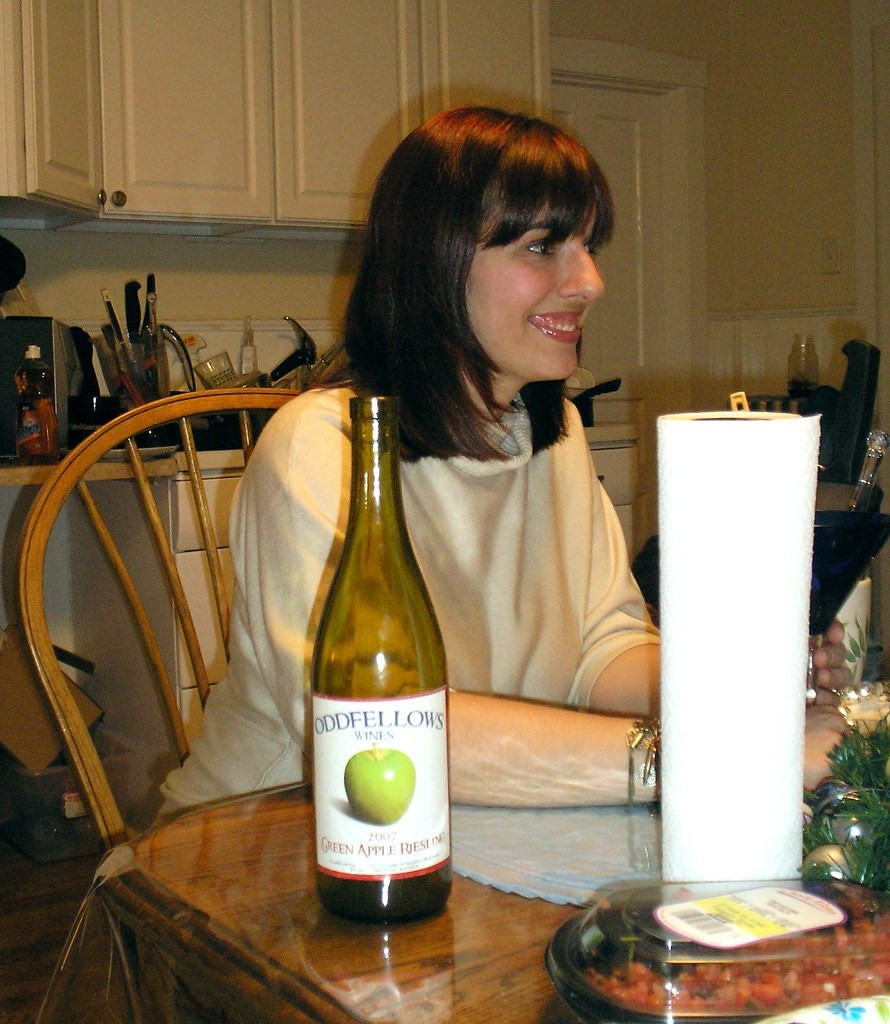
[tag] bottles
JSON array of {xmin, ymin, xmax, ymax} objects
[
  {"xmin": 14, "ymin": 345, "xmax": 59, "ymax": 464},
  {"xmin": 788, "ymin": 333, "xmax": 818, "ymax": 397},
  {"xmin": 312, "ymin": 397, "xmax": 452, "ymax": 926}
]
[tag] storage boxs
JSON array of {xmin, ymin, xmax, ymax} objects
[
  {"xmin": 0, "ymin": 727, "xmax": 136, "ymax": 866},
  {"xmin": 0, "ymin": 625, "xmax": 106, "ymax": 774}
]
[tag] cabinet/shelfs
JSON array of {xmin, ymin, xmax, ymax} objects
[
  {"xmin": 68, "ymin": 441, "xmax": 638, "ymax": 832},
  {"xmin": 1, "ymin": 0, "xmax": 99, "ymax": 231},
  {"xmin": 62, "ymin": 0, "xmax": 553, "ymax": 243}
]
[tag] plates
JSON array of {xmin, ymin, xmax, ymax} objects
[{"xmin": 101, "ymin": 444, "xmax": 180, "ymax": 460}]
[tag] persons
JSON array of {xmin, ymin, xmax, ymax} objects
[{"xmin": 152, "ymin": 105, "xmax": 871, "ymax": 832}]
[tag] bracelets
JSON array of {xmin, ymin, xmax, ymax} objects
[{"xmin": 623, "ymin": 717, "xmax": 664, "ymax": 813}]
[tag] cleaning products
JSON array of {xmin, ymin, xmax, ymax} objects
[{"xmin": 14, "ymin": 345, "xmax": 60, "ymax": 465}]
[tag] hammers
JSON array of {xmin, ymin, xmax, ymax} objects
[{"xmin": 245, "ymin": 316, "xmax": 319, "ymax": 389}]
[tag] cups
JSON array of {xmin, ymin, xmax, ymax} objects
[
  {"xmin": 564, "ymin": 368, "xmax": 595, "ymax": 397},
  {"xmin": 66, "ymin": 395, "xmax": 119, "ymax": 446}
]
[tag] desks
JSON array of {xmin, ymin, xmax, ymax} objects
[{"xmin": 34, "ymin": 782, "xmax": 665, "ymax": 1024}]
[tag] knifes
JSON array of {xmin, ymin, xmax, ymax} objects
[{"xmin": 124, "ymin": 280, "xmax": 141, "ymax": 375}]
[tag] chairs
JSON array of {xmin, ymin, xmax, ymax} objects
[{"xmin": 9, "ymin": 388, "xmax": 316, "ymax": 1024}]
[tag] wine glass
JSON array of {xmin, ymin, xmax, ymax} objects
[{"xmin": 807, "ymin": 510, "xmax": 890, "ymax": 707}]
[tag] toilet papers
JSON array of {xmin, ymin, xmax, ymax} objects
[{"xmin": 655, "ymin": 410, "xmax": 825, "ymax": 884}]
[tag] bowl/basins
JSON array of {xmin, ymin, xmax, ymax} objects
[{"xmin": 546, "ymin": 882, "xmax": 890, "ymax": 1024}]
